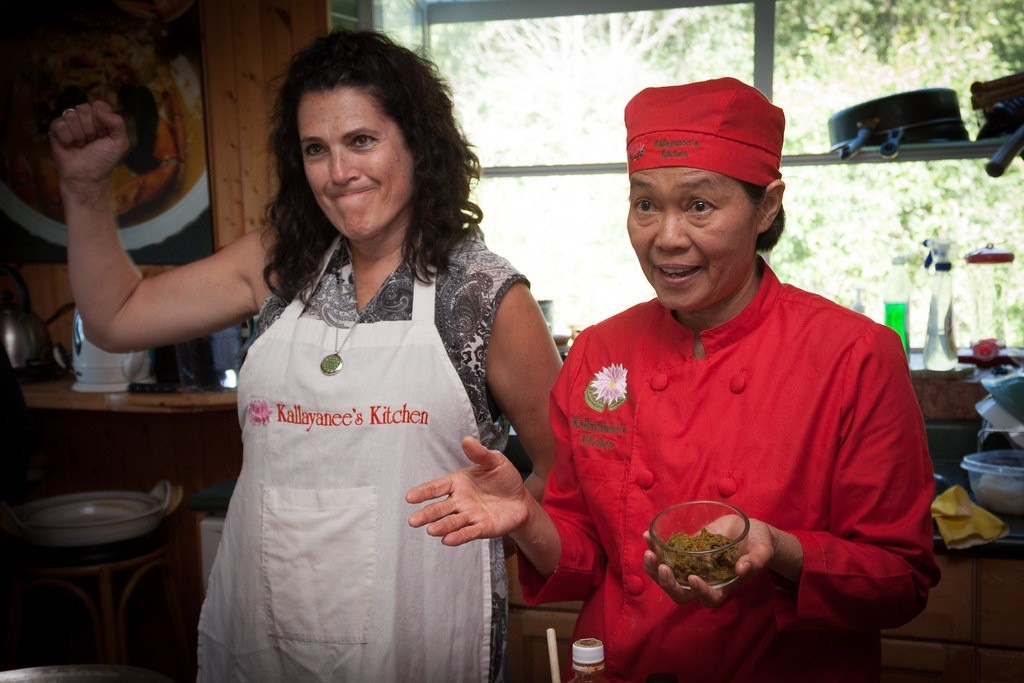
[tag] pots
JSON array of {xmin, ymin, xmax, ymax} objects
[
  {"xmin": 0, "ymin": 480, "xmax": 183, "ymax": 546},
  {"xmin": 830, "ymin": 89, "xmax": 970, "ymax": 163}
]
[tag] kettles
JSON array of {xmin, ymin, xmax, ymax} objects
[{"xmin": 0, "ymin": 263, "xmax": 75, "ymax": 383}]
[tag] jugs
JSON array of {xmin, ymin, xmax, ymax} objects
[{"xmin": 71, "ymin": 273, "xmax": 156, "ymax": 393}]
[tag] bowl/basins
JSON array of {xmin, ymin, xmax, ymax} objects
[
  {"xmin": 650, "ymin": 501, "xmax": 750, "ymax": 590},
  {"xmin": 959, "ymin": 447, "xmax": 1024, "ymax": 516}
]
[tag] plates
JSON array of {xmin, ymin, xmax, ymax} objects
[{"xmin": 2, "ymin": 21, "xmax": 210, "ymax": 250}]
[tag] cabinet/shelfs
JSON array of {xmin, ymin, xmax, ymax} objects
[{"xmin": 192, "ymin": 511, "xmax": 1024, "ymax": 683}]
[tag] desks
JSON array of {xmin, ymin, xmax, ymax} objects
[{"xmin": 20, "ymin": 383, "xmax": 238, "ymax": 415}]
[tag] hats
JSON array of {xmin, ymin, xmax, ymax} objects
[{"xmin": 624, "ymin": 77, "xmax": 785, "ymax": 188}]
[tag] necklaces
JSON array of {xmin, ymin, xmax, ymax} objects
[{"xmin": 319, "ymin": 238, "xmax": 404, "ymax": 377}]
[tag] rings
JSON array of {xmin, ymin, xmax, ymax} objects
[{"xmin": 62, "ymin": 109, "xmax": 75, "ymax": 120}]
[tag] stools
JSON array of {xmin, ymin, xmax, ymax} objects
[{"xmin": 8, "ymin": 530, "xmax": 187, "ymax": 666}]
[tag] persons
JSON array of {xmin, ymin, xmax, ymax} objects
[
  {"xmin": 404, "ymin": 76, "xmax": 942, "ymax": 683},
  {"xmin": 46, "ymin": 25, "xmax": 563, "ymax": 683}
]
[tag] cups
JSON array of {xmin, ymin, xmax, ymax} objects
[{"xmin": 176, "ymin": 316, "xmax": 259, "ymax": 392}]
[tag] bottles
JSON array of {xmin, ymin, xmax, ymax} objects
[
  {"xmin": 884, "ymin": 235, "xmax": 1022, "ymax": 372},
  {"xmin": 566, "ymin": 637, "xmax": 612, "ymax": 683}
]
